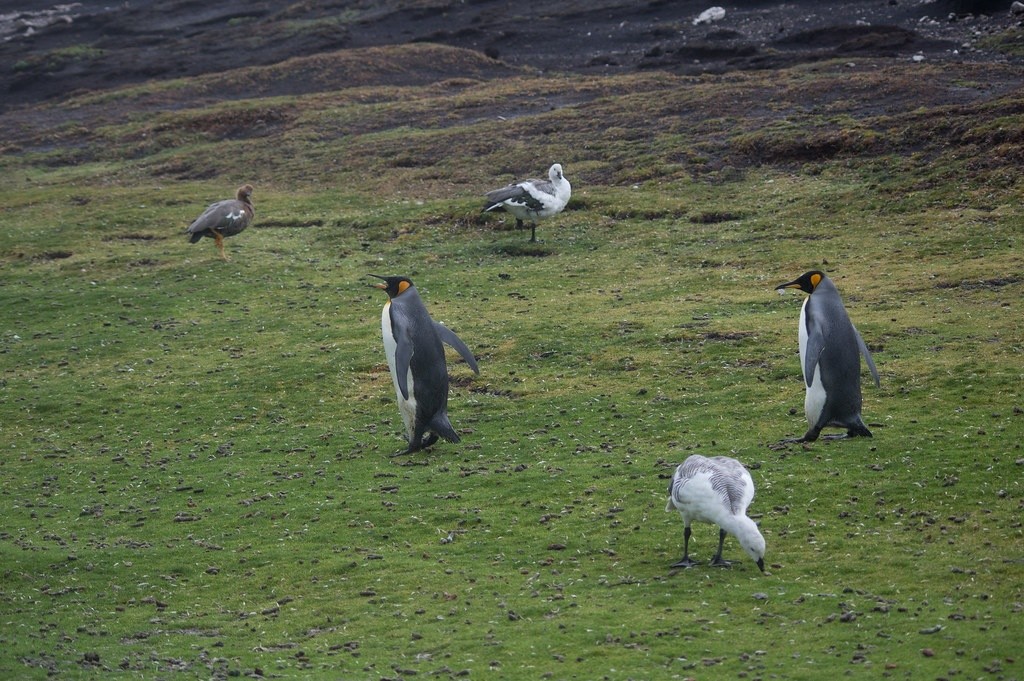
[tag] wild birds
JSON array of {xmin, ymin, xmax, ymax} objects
[
  {"xmin": 664, "ymin": 453, "xmax": 767, "ymax": 574},
  {"xmin": 186, "ymin": 184, "xmax": 255, "ymax": 263},
  {"xmin": 480, "ymin": 164, "xmax": 571, "ymax": 243}
]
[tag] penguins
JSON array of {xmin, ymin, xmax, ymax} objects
[
  {"xmin": 774, "ymin": 270, "xmax": 881, "ymax": 444},
  {"xmin": 361, "ymin": 270, "xmax": 482, "ymax": 456}
]
